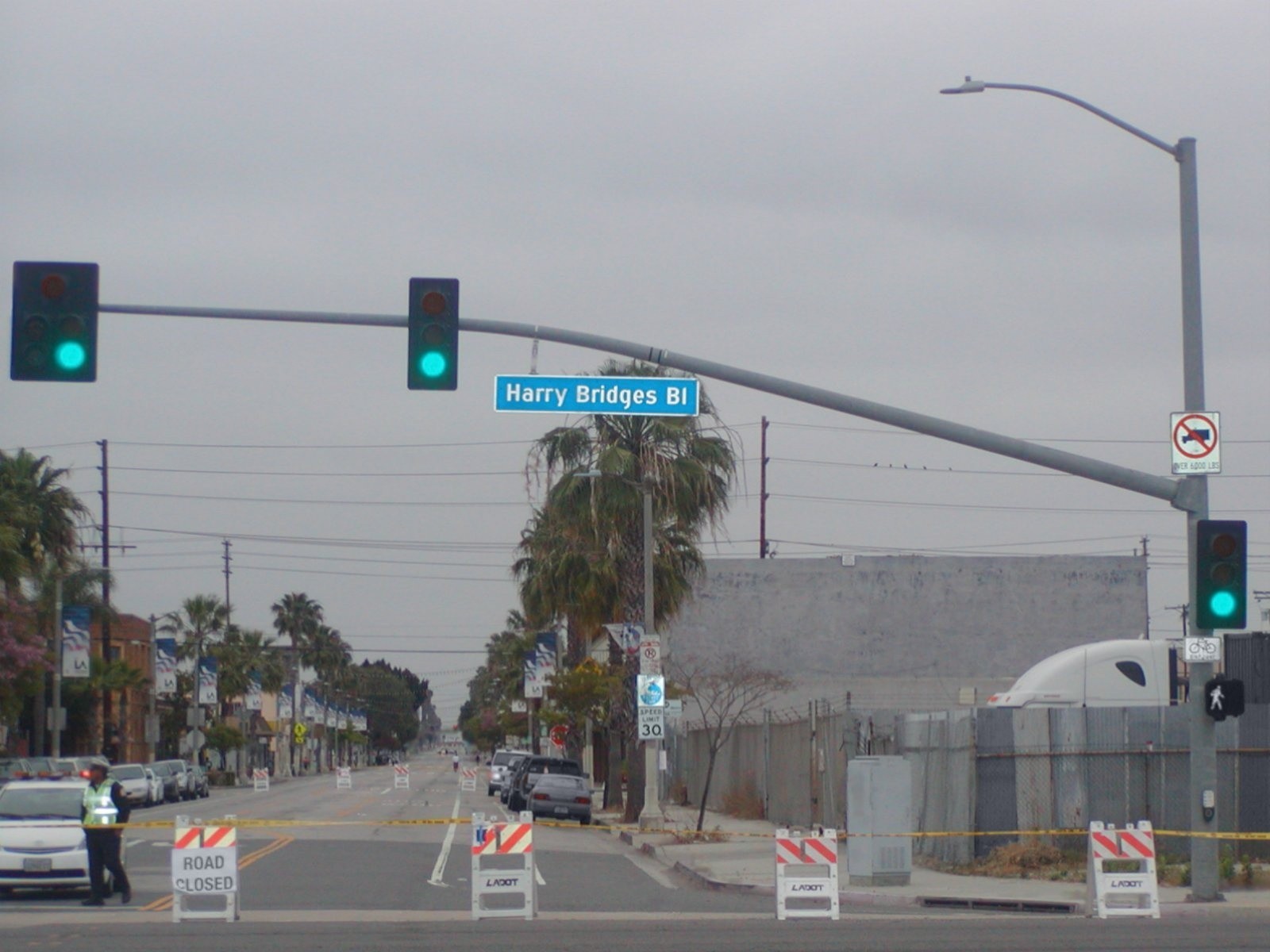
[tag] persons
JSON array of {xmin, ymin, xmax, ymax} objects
[
  {"xmin": 453, "ymin": 753, "xmax": 458, "ymax": 772},
  {"xmin": 303, "ymin": 756, "xmax": 308, "ymax": 769},
  {"xmin": 476, "ymin": 755, "xmax": 479, "ymax": 766},
  {"xmin": 81, "ymin": 759, "xmax": 130, "ymax": 905},
  {"xmin": 204, "ymin": 758, "xmax": 212, "ymax": 769}
]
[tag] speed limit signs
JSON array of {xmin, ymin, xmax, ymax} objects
[{"xmin": 638, "ymin": 707, "xmax": 664, "ymax": 741}]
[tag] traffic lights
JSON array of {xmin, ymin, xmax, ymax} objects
[
  {"xmin": 10, "ymin": 261, "xmax": 99, "ymax": 382},
  {"xmin": 1206, "ymin": 673, "xmax": 1245, "ymax": 721},
  {"xmin": 1196, "ymin": 518, "xmax": 1249, "ymax": 629},
  {"xmin": 409, "ymin": 278, "xmax": 457, "ymax": 390}
]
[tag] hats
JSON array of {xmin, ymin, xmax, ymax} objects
[{"xmin": 84, "ymin": 755, "xmax": 111, "ymax": 770}]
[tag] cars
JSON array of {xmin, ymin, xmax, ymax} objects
[
  {"xmin": 0, "ymin": 770, "xmax": 128, "ymax": 898},
  {"xmin": 485, "ymin": 746, "xmax": 595, "ymax": 826},
  {"xmin": 0, "ymin": 754, "xmax": 211, "ymax": 809}
]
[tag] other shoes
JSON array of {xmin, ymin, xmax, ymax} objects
[
  {"xmin": 122, "ymin": 886, "xmax": 131, "ymax": 904},
  {"xmin": 81, "ymin": 895, "xmax": 105, "ymax": 906}
]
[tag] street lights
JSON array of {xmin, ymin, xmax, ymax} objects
[{"xmin": 944, "ymin": 73, "xmax": 1224, "ymax": 903}]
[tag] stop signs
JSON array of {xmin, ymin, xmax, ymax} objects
[{"xmin": 550, "ymin": 724, "xmax": 569, "ymax": 746}]
